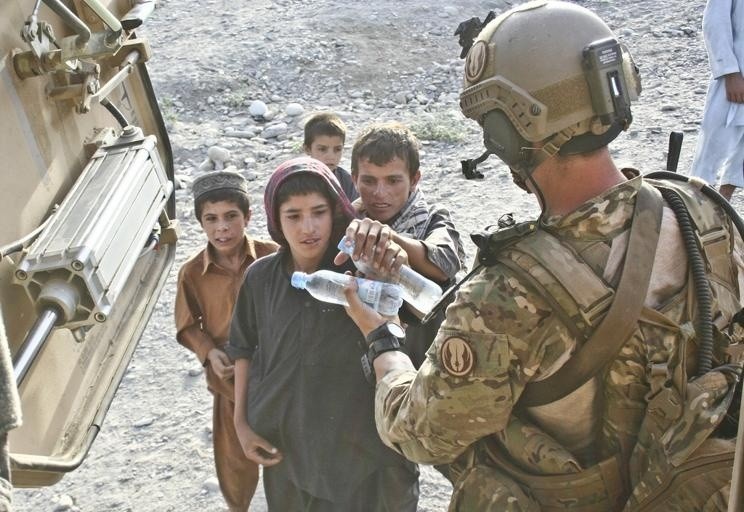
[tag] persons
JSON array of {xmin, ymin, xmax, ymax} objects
[
  {"xmin": 224, "ymin": 155, "xmax": 419, "ymax": 511},
  {"xmin": 173, "ymin": 169, "xmax": 278, "ymax": 512},
  {"xmin": 686, "ymin": 0, "xmax": 744, "ymax": 202},
  {"xmin": 339, "ymin": 0, "xmax": 743, "ymax": 512},
  {"xmin": 334, "ymin": 121, "xmax": 467, "ymax": 371},
  {"xmin": 301, "ymin": 109, "xmax": 361, "ymax": 203}
]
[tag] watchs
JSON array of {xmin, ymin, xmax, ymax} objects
[
  {"xmin": 366, "ymin": 321, "xmax": 407, "ymax": 345},
  {"xmin": 360, "ymin": 335, "xmax": 401, "ymax": 387}
]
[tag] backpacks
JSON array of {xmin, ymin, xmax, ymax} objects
[{"xmin": 621, "ymin": 363, "xmax": 744, "ymax": 512}]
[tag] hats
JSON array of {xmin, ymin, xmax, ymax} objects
[{"xmin": 192, "ymin": 171, "xmax": 248, "ymax": 199}]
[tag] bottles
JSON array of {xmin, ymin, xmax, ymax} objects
[
  {"xmin": 290, "ymin": 270, "xmax": 404, "ymax": 317},
  {"xmin": 336, "ymin": 236, "xmax": 442, "ymax": 314}
]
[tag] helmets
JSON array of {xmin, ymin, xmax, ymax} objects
[{"xmin": 455, "ymin": 0, "xmax": 641, "ymax": 143}]
